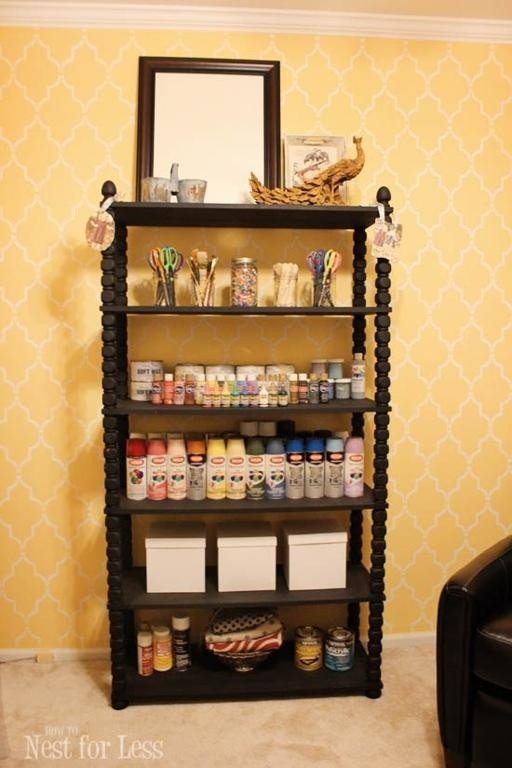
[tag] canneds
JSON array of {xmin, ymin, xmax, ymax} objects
[
  {"xmin": 130, "ymin": 381, "xmax": 153, "ymax": 402},
  {"xmin": 324, "ymin": 626, "xmax": 354, "ymax": 672},
  {"xmin": 130, "ymin": 359, "xmax": 163, "ymax": 381},
  {"xmin": 294, "ymin": 627, "xmax": 324, "ymax": 672}
]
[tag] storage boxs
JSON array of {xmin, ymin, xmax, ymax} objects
[
  {"xmin": 216, "ymin": 520, "xmax": 278, "ymax": 593},
  {"xmin": 279, "ymin": 519, "xmax": 349, "ymax": 591},
  {"xmin": 143, "ymin": 520, "xmax": 207, "ymax": 594}
]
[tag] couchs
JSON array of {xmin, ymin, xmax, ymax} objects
[
  {"xmin": 436, "ymin": 534, "xmax": 512, "ymax": 768},
  {"xmin": 134, "ymin": 56, "xmax": 281, "ymax": 204}
]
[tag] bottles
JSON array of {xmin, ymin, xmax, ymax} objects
[
  {"xmin": 206, "ymin": 373, "xmax": 217, "ymax": 403},
  {"xmin": 327, "ymin": 358, "xmax": 345, "ymax": 378},
  {"xmin": 174, "ymin": 361, "xmax": 204, "ymax": 381},
  {"xmin": 205, "ymin": 363, "xmax": 235, "ymax": 373},
  {"xmin": 288, "ymin": 373, "xmax": 298, "ymax": 404},
  {"xmin": 257, "ymin": 373, "xmax": 267, "ymax": 404},
  {"xmin": 152, "ymin": 626, "xmax": 172, "ymax": 673},
  {"xmin": 309, "ymin": 373, "xmax": 319, "ymax": 403},
  {"xmin": 236, "ymin": 373, "xmax": 247, "ymax": 404},
  {"xmin": 278, "ymin": 384, "xmax": 289, "ymax": 406},
  {"xmin": 222, "ymin": 381, "xmax": 230, "ymax": 407},
  {"xmin": 351, "ymin": 352, "xmax": 366, "ymax": 398},
  {"xmin": 226, "ymin": 373, "xmax": 236, "ymax": 404},
  {"xmin": 195, "ymin": 373, "xmax": 205, "ymax": 404},
  {"xmin": 185, "ymin": 373, "xmax": 195, "ymax": 404},
  {"xmin": 311, "ymin": 358, "xmax": 328, "ymax": 377},
  {"xmin": 217, "ymin": 373, "xmax": 226, "ymax": 404},
  {"xmin": 298, "ymin": 373, "xmax": 309, "ymax": 404},
  {"xmin": 259, "ymin": 386, "xmax": 269, "ymax": 407},
  {"xmin": 266, "ymin": 362, "xmax": 296, "ymax": 373},
  {"xmin": 279, "ymin": 372, "xmax": 290, "ymax": 402},
  {"xmin": 235, "ymin": 363, "xmax": 266, "ymax": 374},
  {"xmin": 231, "ymin": 386, "xmax": 240, "ymax": 406},
  {"xmin": 241, "ymin": 385, "xmax": 249, "ymax": 406},
  {"xmin": 267, "ymin": 373, "xmax": 279, "ymax": 404},
  {"xmin": 335, "ymin": 378, "xmax": 351, "ymax": 398},
  {"xmin": 318, "ymin": 373, "xmax": 330, "ymax": 403},
  {"xmin": 203, "ymin": 382, "xmax": 211, "ymax": 407},
  {"xmin": 230, "ymin": 257, "xmax": 258, "ymax": 305},
  {"xmin": 247, "ymin": 373, "xmax": 257, "ymax": 404},
  {"xmin": 250, "ymin": 386, "xmax": 259, "ymax": 407},
  {"xmin": 327, "ymin": 378, "xmax": 335, "ymax": 398},
  {"xmin": 212, "ymin": 381, "xmax": 221, "ymax": 407},
  {"xmin": 171, "ymin": 613, "xmax": 191, "ymax": 672},
  {"xmin": 174, "ymin": 373, "xmax": 184, "ymax": 404},
  {"xmin": 152, "ymin": 373, "xmax": 163, "ymax": 403},
  {"xmin": 269, "ymin": 385, "xmax": 278, "ymax": 406},
  {"xmin": 164, "ymin": 373, "xmax": 173, "ymax": 404}
]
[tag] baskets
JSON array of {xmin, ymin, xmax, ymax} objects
[{"xmin": 202, "ymin": 608, "xmax": 275, "ymax": 672}]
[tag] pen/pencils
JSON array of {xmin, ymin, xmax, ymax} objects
[{"xmin": 186, "ymin": 249, "xmax": 221, "ymax": 307}]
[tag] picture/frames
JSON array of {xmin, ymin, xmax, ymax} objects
[{"xmin": 281, "ymin": 133, "xmax": 348, "ymax": 206}]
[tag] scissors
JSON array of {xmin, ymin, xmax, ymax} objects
[
  {"xmin": 147, "ymin": 246, "xmax": 184, "ymax": 306},
  {"xmin": 305, "ymin": 248, "xmax": 343, "ymax": 307}
]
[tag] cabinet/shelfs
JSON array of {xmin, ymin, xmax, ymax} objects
[{"xmin": 99, "ymin": 180, "xmax": 393, "ymax": 710}]
